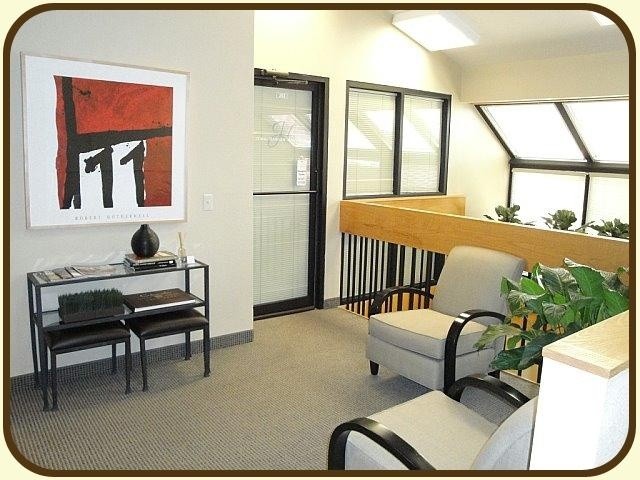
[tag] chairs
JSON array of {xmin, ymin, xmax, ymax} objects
[
  {"xmin": 328, "ymin": 372, "xmax": 539, "ymax": 471},
  {"xmin": 367, "ymin": 242, "xmax": 524, "ymax": 391}
]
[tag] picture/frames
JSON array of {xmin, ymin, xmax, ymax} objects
[{"xmin": 20, "ymin": 51, "xmax": 190, "ymax": 231}]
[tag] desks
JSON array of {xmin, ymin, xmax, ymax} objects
[{"xmin": 27, "ymin": 258, "xmax": 218, "ymax": 411}]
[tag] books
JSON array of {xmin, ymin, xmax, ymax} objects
[
  {"xmin": 121, "ymin": 288, "xmax": 197, "ymax": 313},
  {"xmin": 33, "ymin": 249, "xmax": 179, "ymax": 284}
]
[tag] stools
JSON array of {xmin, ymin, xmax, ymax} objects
[{"xmin": 43, "ymin": 309, "xmax": 209, "ymax": 411}]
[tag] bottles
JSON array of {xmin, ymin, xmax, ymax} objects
[{"xmin": 176, "ymin": 232, "xmax": 188, "ymax": 267}]
